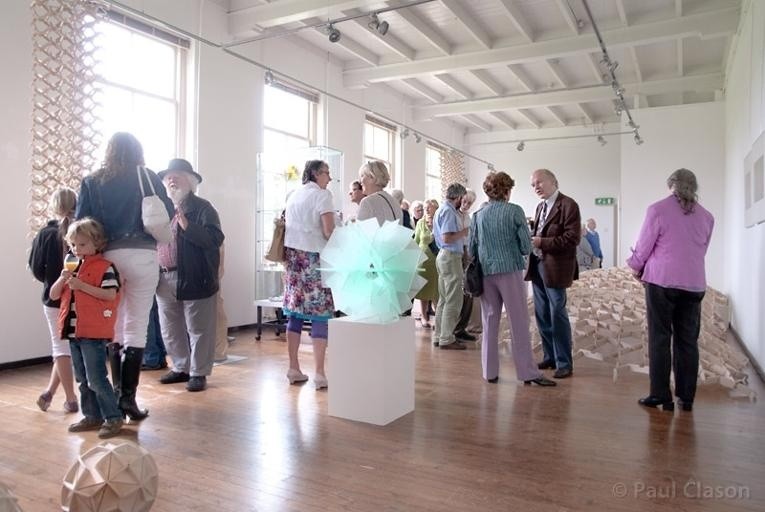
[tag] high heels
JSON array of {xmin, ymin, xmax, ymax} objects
[
  {"xmin": 314, "ymin": 373, "xmax": 328, "ymax": 390},
  {"xmin": 287, "ymin": 369, "xmax": 308, "ymax": 384},
  {"xmin": 677, "ymin": 398, "xmax": 693, "ymax": 411},
  {"xmin": 639, "ymin": 396, "xmax": 674, "ymax": 411}
]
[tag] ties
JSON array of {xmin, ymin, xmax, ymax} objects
[{"xmin": 533, "ymin": 202, "xmax": 547, "ymax": 260}]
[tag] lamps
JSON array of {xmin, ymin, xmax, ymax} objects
[
  {"xmin": 328, "ymin": 13, "xmax": 389, "ymax": 42},
  {"xmin": 605, "ymin": 60, "xmax": 644, "ymax": 145},
  {"xmin": 517, "ymin": 142, "xmax": 525, "ymax": 151},
  {"xmin": 400, "ymin": 129, "xmax": 496, "ymax": 174},
  {"xmin": 598, "ymin": 136, "xmax": 605, "ymax": 144}
]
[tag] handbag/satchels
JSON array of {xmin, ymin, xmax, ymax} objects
[
  {"xmin": 266, "ymin": 218, "xmax": 285, "ymax": 261},
  {"xmin": 141, "ymin": 196, "xmax": 172, "ymax": 242},
  {"xmin": 464, "ymin": 262, "xmax": 484, "ymax": 297}
]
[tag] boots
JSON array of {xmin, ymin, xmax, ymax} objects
[
  {"xmin": 108, "ymin": 342, "xmax": 124, "ymax": 400},
  {"xmin": 117, "ymin": 346, "xmax": 148, "ymax": 420}
]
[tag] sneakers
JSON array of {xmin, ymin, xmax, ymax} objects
[{"xmin": 38, "ymin": 362, "xmax": 206, "ymax": 438}]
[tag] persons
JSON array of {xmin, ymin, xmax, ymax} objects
[
  {"xmin": 47, "ymin": 215, "xmax": 125, "ymax": 438},
  {"xmin": 575, "ymin": 224, "xmax": 601, "ymax": 274},
  {"xmin": 138, "ymin": 158, "xmax": 229, "ymax": 393},
  {"xmin": 584, "ymin": 218, "xmax": 603, "ymax": 268},
  {"xmin": 523, "ymin": 167, "xmax": 582, "ymax": 380},
  {"xmin": 281, "ymin": 157, "xmax": 337, "ymax": 391},
  {"xmin": 26, "ymin": 188, "xmax": 79, "ymax": 413},
  {"xmin": 75, "ymin": 130, "xmax": 176, "ymax": 421},
  {"xmin": 466, "ymin": 170, "xmax": 557, "ymax": 388},
  {"xmin": 347, "ymin": 159, "xmax": 483, "ymax": 351},
  {"xmin": 625, "ymin": 165, "xmax": 714, "ymax": 413}
]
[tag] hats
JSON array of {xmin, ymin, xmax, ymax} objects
[{"xmin": 157, "ymin": 159, "xmax": 202, "ymax": 183}]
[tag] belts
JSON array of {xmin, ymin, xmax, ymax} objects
[{"xmin": 160, "ymin": 265, "xmax": 177, "ymax": 273}]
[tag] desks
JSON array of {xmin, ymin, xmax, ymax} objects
[{"xmin": 253, "ymin": 296, "xmax": 340, "ymax": 340}]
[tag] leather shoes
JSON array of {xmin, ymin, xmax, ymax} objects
[{"xmin": 402, "ymin": 311, "xmax": 571, "ymax": 386}]
